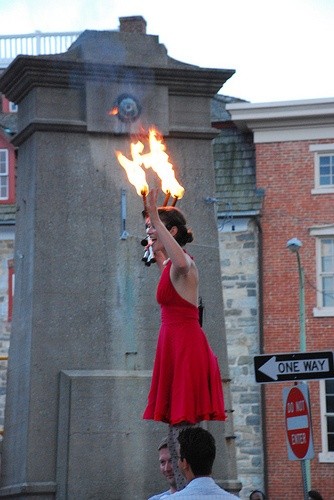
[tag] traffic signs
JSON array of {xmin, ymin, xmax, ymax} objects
[{"xmin": 253, "ymin": 350, "xmax": 334, "ymax": 383}]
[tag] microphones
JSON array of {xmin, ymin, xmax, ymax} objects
[{"xmin": 141, "ymin": 236, "xmax": 149, "ymax": 246}]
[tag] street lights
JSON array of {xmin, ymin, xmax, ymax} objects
[{"xmin": 283, "ymin": 236, "xmax": 314, "ymax": 500}]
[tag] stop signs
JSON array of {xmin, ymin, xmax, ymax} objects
[{"xmin": 281, "ymin": 383, "xmax": 315, "ymax": 460}]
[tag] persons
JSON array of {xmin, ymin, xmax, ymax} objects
[
  {"xmin": 147, "ymin": 427, "xmax": 324, "ymax": 500},
  {"xmin": 141, "ymin": 189, "xmax": 225, "ymax": 491}
]
